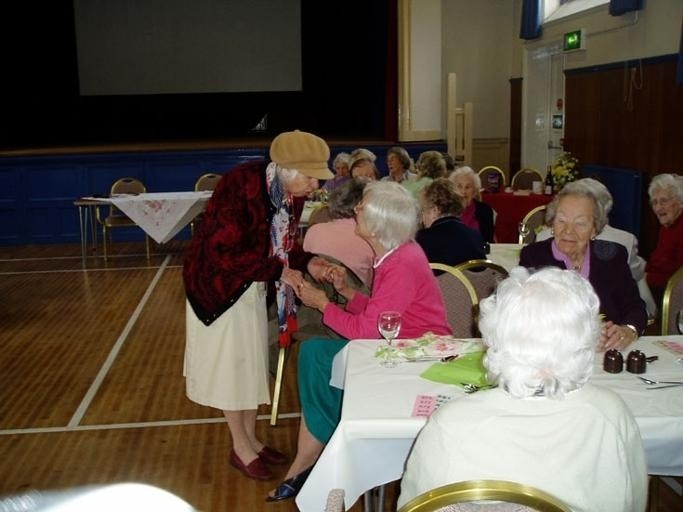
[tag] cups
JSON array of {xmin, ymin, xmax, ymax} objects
[
  {"xmin": 604, "ymin": 349, "xmax": 622, "ymax": 374},
  {"xmin": 627, "ymin": 350, "xmax": 646, "ymax": 374},
  {"xmin": 533, "ymin": 181, "xmax": 544, "ymax": 195}
]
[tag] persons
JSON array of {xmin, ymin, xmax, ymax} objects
[
  {"xmin": 265, "ymin": 147, "xmax": 682, "ymax": 512},
  {"xmin": 183, "ymin": 129, "xmax": 336, "ymax": 481}
]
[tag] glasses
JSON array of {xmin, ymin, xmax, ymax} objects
[
  {"xmin": 353, "ymin": 200, "xmax": 363, "ymax": 214},
  {"xmin": 650, "ymin": 195, "xmax": 677, "ymax": 205}
]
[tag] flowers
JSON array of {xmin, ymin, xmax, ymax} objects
[{"xmin": 550, "ymin": 151, "xmax": 579, "ymax": 191}]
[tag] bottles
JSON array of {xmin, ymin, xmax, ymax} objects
[{"xmin": 544, "ymin": 165, "xmax": 554, "ymax": 195}]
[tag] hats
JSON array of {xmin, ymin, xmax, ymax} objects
[
  {"xmin": 269, "ymin": 130, "xmax": 335, "ymax": 180},
  {"xmin": 349, "ymin": 148, "xmax": 376, "ymax": 170}
]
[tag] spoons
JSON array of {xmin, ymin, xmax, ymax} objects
[
  {"xmin": 636, "ymin": 376, "xmax": 683, "ymax": 387},
  {"xmin": 458, "ymin": 383, "xmax": 498, "ymax": 393},
  {"xmin": 405, "ymin": 353, "xmax": 458, "ymax": 362}
]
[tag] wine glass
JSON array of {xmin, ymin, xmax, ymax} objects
[{"xmin": 376, "ymin": 312, "xmax": 402, "ymax": 368}]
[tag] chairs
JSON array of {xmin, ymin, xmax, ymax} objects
[
  {"xmin": 191, "ymin": 173, "xmax": 224, "ymax": 237},
  {"xmin": 96, "ymin": 177, "xmax": 150, "ymax": 261}
]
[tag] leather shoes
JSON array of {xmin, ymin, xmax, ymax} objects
[
  {"xmin": 265, "ymin": 463, "xmax": 315, "ymax": 502},
  {"xmin": 257, "ymin": 444, "xmax": 289, "ymax": 465},
  {"xmin": 228, "ymin": 447, "xmax": 277, "ymax": 482}
]
